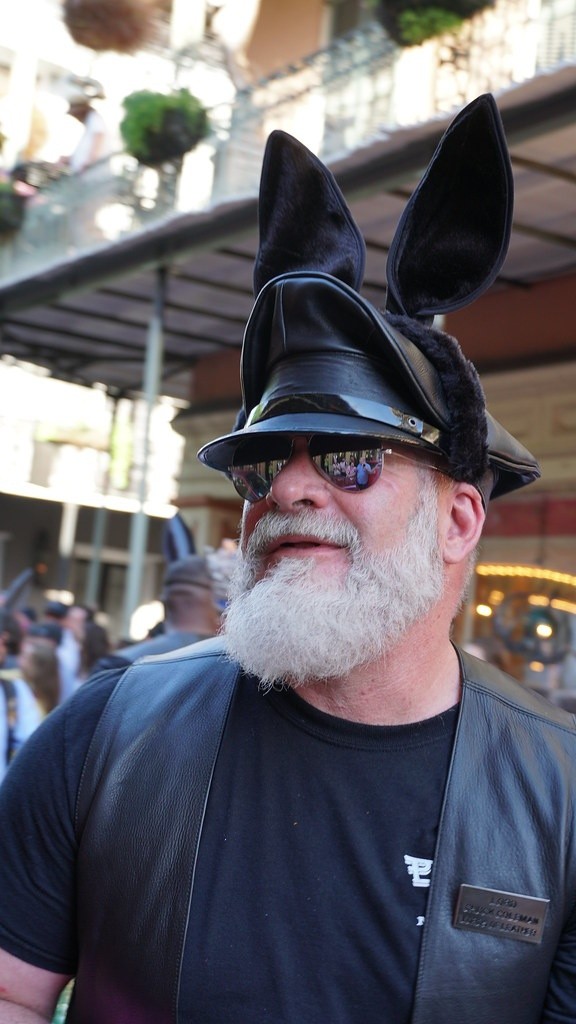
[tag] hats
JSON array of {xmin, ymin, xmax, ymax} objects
[{"xmin": 196, "ymin": 270, "xmax": 542, "ymax": 500}]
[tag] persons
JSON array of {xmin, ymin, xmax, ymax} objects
[
  {"xmin": 89, "ymin": 553, "xmax": 231, "ymax": 676},
  {"xmin": 0, "ymin": 92, "xmax": 576, "ymax": 1024},
  {"xmin": 0, "ymin": 600, "xmax": 108, "ymax": 773},
  {"xmin": 333, "ymin": 455, "xmax": 356, "ymax": 479},
  {"xmin": 357, "ymin": 456, "xmax": 371, "ymax": 490}
]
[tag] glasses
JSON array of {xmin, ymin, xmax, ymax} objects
[{"xmin": 226, "ymin": 436, "xmax": 447, "ymax": 504}]
